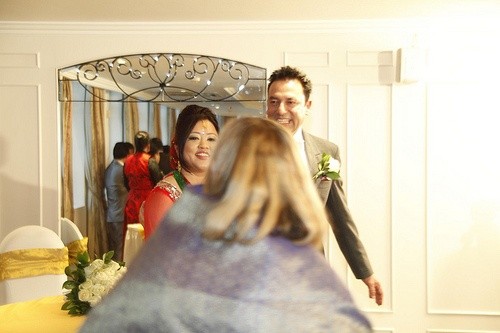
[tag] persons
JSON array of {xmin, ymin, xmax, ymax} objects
[
  {"xmin": 103, "ymin": 131, "xmax": 176, "ymax": 263},
  {"xmin": 143, "ymin": 104, "xmax": 221, "ymax": 241},
  {"xmin": 265, "ymin": 66, "xmax": 384, "ymax": 306},
  {"xmin": 75, "ymin": 116, "xmax": 375, "ymax": 333}
]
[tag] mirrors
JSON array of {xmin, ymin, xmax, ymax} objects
[{"xmin": 58, "ymin": 53, "xmax": 267, "ymax": 262}]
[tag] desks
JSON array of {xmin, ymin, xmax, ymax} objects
[{"xmin": 123, "ymin": 223, "xmax": 145, "ymax": 267}]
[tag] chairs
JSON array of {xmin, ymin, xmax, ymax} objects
[
  {"xmin": 61, "ymin": 218, "xmax": 90, "ymax": 264},
  {"xmin": 0, "ymin": 225, "xmax": 72, "ymax": 306}
]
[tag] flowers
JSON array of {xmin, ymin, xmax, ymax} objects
[
  {"xmin": 311, "ymin": 152, "xmax": 341, "ymax": 183},
  {"xmin": 61, "ymin": 248, "xmax": 128, "ymax": 314}
]
